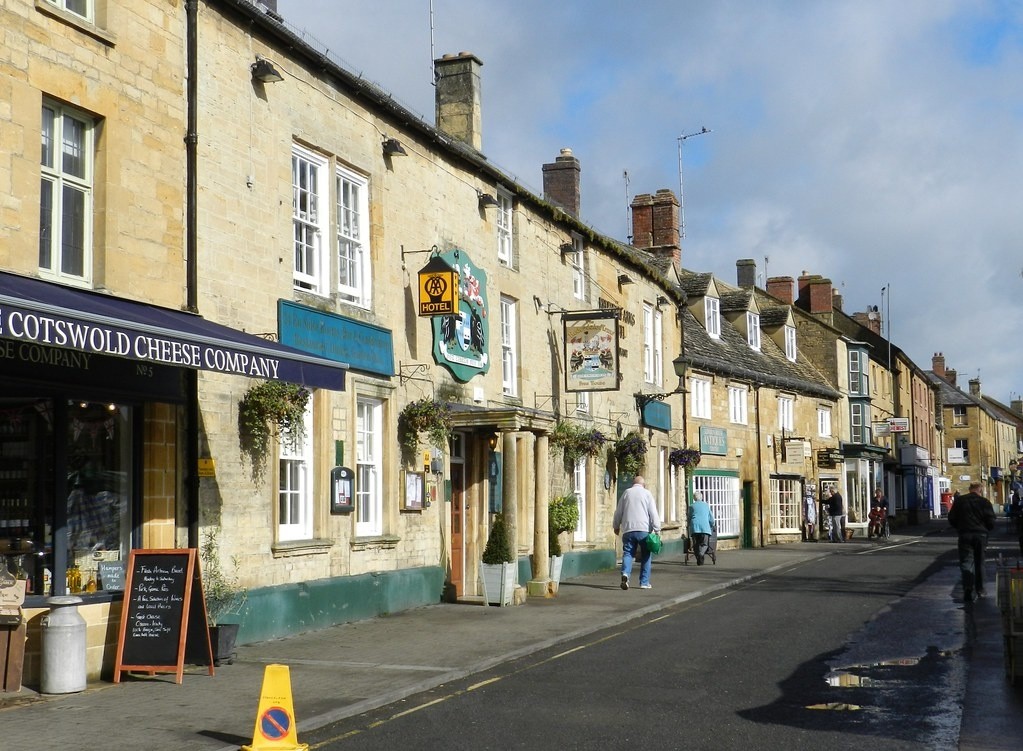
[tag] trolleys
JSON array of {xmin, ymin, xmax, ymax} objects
[{"xmin": 685, "ymin": 525, "xmax": 719, "ymax": 567}]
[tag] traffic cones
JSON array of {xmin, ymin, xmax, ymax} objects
[{"xmin": 238, "ymin": 662, "xmax": 308, "ymax": 751}]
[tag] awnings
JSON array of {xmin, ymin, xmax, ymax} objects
[{"xmin": 0, "ymin": 268, "xmax": 350, "ymax": 394}]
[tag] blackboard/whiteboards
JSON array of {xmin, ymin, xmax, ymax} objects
[
  {"xmin": 115, "ymin": 548, "xmax": 213, "ymax": 671},
  {"xmin": 98, "ymin": 562, "xmax": 126, "ymax": 590}
]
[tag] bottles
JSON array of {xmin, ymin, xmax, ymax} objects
[
  {"xmin": 1, "ymin": 408, "xmax": 32, "ymax": 552},
  {"xmin": 1, "ymin": 557, "xmax": 26, "ymax": 580},
  {"xmin": 66, "ymin": 565, "xmax": 96, "ymax": 594}
]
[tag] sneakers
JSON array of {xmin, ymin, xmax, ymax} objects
[
  {"xmin": 620, "ymin": 574, "xmax": 629, "ymax": 590},
  {"xmin": 640, "ymin": 584, "xmax": 652, "ymax": 588}
]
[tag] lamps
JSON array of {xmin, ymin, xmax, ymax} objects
[
  {"xmin": 252, "ymin": 60, "xmax": 283, "ymax": 82},
  {"xmin": 561, "ymin": 243, "xmax": 576, "ymax": 254},
  {"xmin": 619, "ymin": 275, "xmax": 635, "ymax": 285},
  {"xmin": 634, "ymin": 353, "xmax": 691, "ymax": 408},
  {"xmin": 382, "ymin": 139, "xmax": 407, "ymax": 156},
  {"xmin": 478, "ymin": 194, "xmax": 500, "ymax": 208},
  {"xmin": 657, "ymin": 296, "xmax": 669, "ymax": 306}
]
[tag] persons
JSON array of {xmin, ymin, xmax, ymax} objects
[
  {"xmin": 686, "ymin": 490, "xmax": 714, "ymax": 566},
  {"xmin": 813, "ymin": 484, "xmax": 846, "ymax": 545},
  {"xmin": 952, "ymin": 489, "xmax": 961, "ymax": 500},
  {"xmin": 867, "ymin": 487, "xmax": 890, "ymax": 538},
  {"xmin": 948, "ymin": 483, "xmax": 996, "ymax": 609},
  {"xmin": 612, "ymin": 476, "xmax": 660, "ymax": 590},
  {"xmin": 1008, "ymin": 490, "xmax": 1014, "ymax": 505}
]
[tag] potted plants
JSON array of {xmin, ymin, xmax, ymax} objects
[
  {"xmin": 670, "ymin": 447, "xmax": 701, "ymax": 476},
  {"xmin": 614, "ymin": 430, "xmax": 650, "ymax": 474},
  {"xmin": 550, "ymin": 422, "xmax": 604, "ymax": 464},
  {"xmin": 398, "ymin": 398, "xmax": 457, "ymax": 454},
  {"xmin": 480, "ymin": 514, "xmax": 516, "ymax": 608},
  {"xmin": 530, "ymin": 493, "xmax": 580, "ymax": 591},
  {"xmin": 198, "ymin": 500, "xmax": 248, "ymax": 659},
  {"xmin": 241, "ymin": 379, "xmax": 311, "ymax": 455}
]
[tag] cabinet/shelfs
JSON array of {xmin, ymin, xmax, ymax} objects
[{"xmin": 0, "ymin": 395, "xmax": 54, "ymax": 554}]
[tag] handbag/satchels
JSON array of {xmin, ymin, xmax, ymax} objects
[{"xmin": 646, "ymin": 533, "xmax": 662, "ymax": 554}]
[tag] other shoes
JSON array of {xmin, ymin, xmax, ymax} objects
[
  {"xmin": 978, "ymin": 590, "xmax": 988, "ymax": 597},
  {"xmin": 964, "ymin": 586, "xmax": 973, "ymax": 601},
  {"xmin": 697, "ymin": 554, "xmax": 704, "ymax": 565}
]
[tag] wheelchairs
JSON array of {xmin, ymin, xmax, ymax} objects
[{"xmin": 868, "ymin": 507, "xmax": 890, "ymax": 540}]
